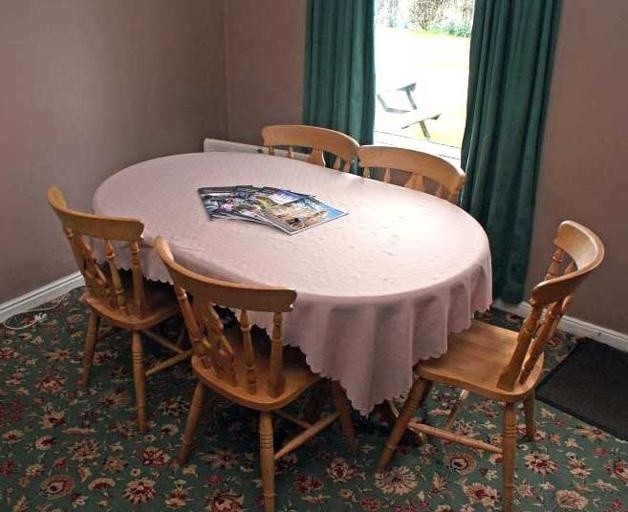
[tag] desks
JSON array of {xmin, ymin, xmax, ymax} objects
[{"xmin": 92, "ymin": 151, "xmax": 493, "ymax": 447}]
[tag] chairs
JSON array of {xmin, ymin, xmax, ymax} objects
[
  {"xmin": 358, "ymin": 144, "xmax": 466, "ymax": 202},
  {"xmin": 154, "ymin": 236, "xmax": 356, "ymax": 512},
  {"xmin": 46, "ymin": 185, "xmax": 193, "ymax": 435},
  {"xmin": 261, "ymin": 124, "xmax": 360, "ymax": 173},
  {"xmin": 377, "ymin": 220, "xmax": 606, "ymax": 512}
]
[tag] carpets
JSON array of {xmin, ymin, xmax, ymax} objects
[{"xmin": 534, "ymin": 336, "xmax": 628, "ymax": 442}]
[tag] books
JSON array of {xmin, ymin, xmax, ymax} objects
[{"xmin": 198, "ymin": 184, "xmax": 348, "ymax": 235}]
